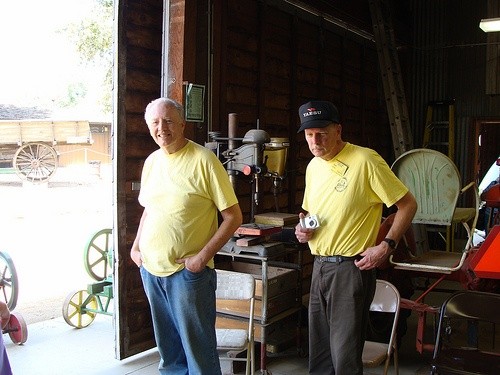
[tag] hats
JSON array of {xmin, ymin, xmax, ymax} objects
[{"xmin": 297, "ymin": 101, "xmax": 339, "ymax": 133}]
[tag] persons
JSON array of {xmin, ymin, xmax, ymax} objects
[
  {"xmin": 128, "ymin": 98, "xmax": 243, "ymax": 375},
  {"xmin": 295, "ymin": 100, "xmax": 418, "ymax": 375}
]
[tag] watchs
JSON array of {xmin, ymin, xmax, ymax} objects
[{"xmin": 384, "ymin": 238, "xmax": 396, "ymax": 249}]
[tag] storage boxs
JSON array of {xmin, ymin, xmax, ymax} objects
[{"xmin": 215, "ymin": 260, "xmax": 298, "ymax": 343}]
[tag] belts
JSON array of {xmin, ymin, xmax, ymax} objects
[{"xmin": 315, "ymin": 256, "xmax": 353, "ymax": 263}]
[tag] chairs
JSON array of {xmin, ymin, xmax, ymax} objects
[
  {"xmin": 430, "ymin": 290, "xmax": 500, "ymax": 375},
  {"xmin": 389, "ymin": 148, "xmax": 481, "ymax": 271},
  {"xmin": 212, "ymin": 269, "xmax": 256, "ymax": 375},
  {"xmin": 362, "ymin": 279, "xmax": 401, "ymax": 375}
]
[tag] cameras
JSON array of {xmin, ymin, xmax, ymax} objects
[{"xmin": 299, "ymin": 214, "xmax": 320, "ymax": 230}]
